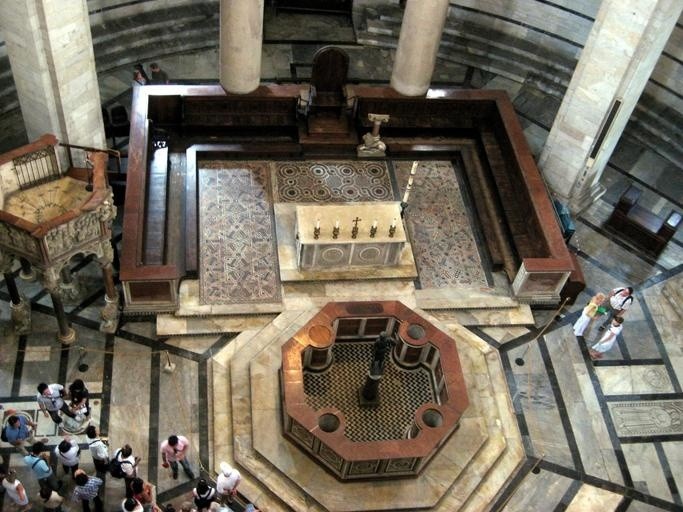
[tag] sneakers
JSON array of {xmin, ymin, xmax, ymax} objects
[
  {"xmin": 75, "ymin": 416, "xmax": 81, "ymax": 421},
  {"xmin": 59, "ymin": 422, "xmax": 64, "ymax": 428}
]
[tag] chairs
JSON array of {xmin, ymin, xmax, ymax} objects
[{"xmin": 297, "ymin": 46, "xmax": 358, "ymax": 137}]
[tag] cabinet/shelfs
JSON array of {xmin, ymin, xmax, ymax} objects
[
  {"xmin": 560, "ymin": 248, "xmax": 585, "ymax": 305},
  {"xmin": 601, "ymin": 184, "xmax": 683, "ymax": 260}
]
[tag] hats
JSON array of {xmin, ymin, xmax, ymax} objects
[
  {"xmin": 220, "ymin": 462, "xmax": 232, "ymax": 477},
  {"xmin": 182, "ymin": 502, "xmax": 192, "ymax": 510}
]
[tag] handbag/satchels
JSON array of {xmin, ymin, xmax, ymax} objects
[{"xmin": 76, "ymin": 404, "xmax": 87, "ymax": 414}]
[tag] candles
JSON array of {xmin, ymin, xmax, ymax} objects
[
  {"xmin": 392, "ymin": 217, "xmax": 398, "ymax": 228},
  {"xmin": 316, "ymin": 219, "xmax": 320, "ymax": 230},
  {"xmin": 373, "ymin": 219, "xmax": 378, "ymax": 228},
  {"xmin": 335, "ymin": 220, "xmax": 339, "ymax": 228}
]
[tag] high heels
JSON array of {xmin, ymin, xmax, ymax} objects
[{"xmin": 23, "ymin": 503, "xmax": 32, "ymax": 512}]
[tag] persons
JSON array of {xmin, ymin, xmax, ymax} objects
[
  {"xmin": 0, "ymin": 377, "xmax": 261, "ymax": 512},
  {"xmin": 131, "ymin": 62, "xmax": 151, "ymax": 88},
  {"xmin": 146, "ymin": 62, "xmax": 169, "ymax": 85},
  {"xmin": 594, "ymin": 287, "xmax": 634, "ymax": 332},
  {"xmin": 372, "ymin": 330, "xmax": 387, "ymax": 371},
  {"xmin": 571, "ymin": 293, "xmax": 606, "ymax": 338},
  {"xmin": 588, "ymin": 315, "xmax": 624, "ymax": 360}
]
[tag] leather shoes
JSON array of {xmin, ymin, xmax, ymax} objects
[
  {"xmin": 173, "ymin": 472, "xmax": 178, "ymax": 479},
  {"xmin": 58, "ymin": 480, "xmax": 63, "ymax": 490},
  {"xmin": 188, "ymin": 472, "xmax": 194, "ymax": 479}
]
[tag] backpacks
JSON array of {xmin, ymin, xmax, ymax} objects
[
  {"xmin": 615, "ymin": 289, "xmax": 633, "ymax": 306},
  {"xmin": 108, "ymin": 450, "xmax": 132, "ymax": 479},
  {"xmin": 194, "ymin": 487, "xmax": 212, "ymax": 508},
  {"xmin": 1, "ymin": 426, "xmax": 8, "ymax": 442}
]
